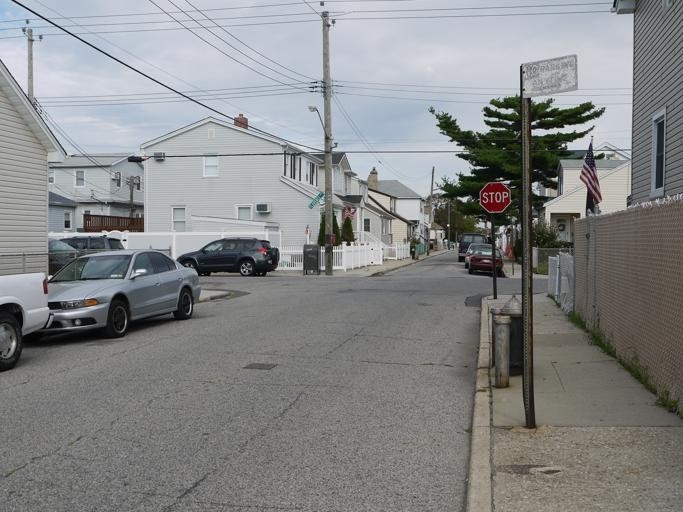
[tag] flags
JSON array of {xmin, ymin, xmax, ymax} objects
[{"xmin": 579, "ymin": 143, "xmax": 602, "ymax": 206}]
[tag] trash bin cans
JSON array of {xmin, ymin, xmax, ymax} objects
[
  {"xmin": 490, "ymin": 308, "xmax": 524, "ymax": 377},
  {"xmin": 303, "ymin": 244, "xmax": 322, "ymax": 275}
]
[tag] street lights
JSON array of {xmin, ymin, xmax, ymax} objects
[{"xmin": 308, "ymin": 105, "xmax": 335, "ymax": 274}]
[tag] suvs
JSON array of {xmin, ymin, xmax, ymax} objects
[
  {"xmin": 458, "ymin": 231, "xmax": 488, "ymax": 262},
  {"xmin": 176, "ymin": 237, "xmax": 280, "ymax": 276}
]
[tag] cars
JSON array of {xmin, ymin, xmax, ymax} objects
[
  {"xmin": 465, "ymin": 242, "xmax": 496, "ymax": 268},
  {"xmin": 468, "ymin": 245, "xmax": 503, "ymax": 275},
  {"xmin": 38, "ymin": 249, "xmax": 200, "ymax": 337},
  {"xmin": 47, "ymin": 237, "xmax": 79, "ymax": 274}
]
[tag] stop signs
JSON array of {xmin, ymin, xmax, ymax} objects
[{"xmin": 479, "ymin": 181, "xmax": 511, "ymax": 214}]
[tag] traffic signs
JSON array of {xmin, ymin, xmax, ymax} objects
[{"xmin": 309, "ymin": 191, "xmax": 324, "ymax": 209}]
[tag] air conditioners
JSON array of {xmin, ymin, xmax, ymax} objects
[
  {"xmin": 154, "ymin": 152, "xmax": 165, "ymax": 160},
  {"xmin": 111, "ymin": 172, "xmax": 119, "ymax": 179},
  {"xmin": 255, "ymin": 202, "xmax": 271, "ymax": 213}
]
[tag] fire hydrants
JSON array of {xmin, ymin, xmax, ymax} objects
[{"xmin": 490, "ymin": 293, "xmax": 523, "ymax": 376}]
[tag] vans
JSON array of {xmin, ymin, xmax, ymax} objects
[{"xmin": 48, "ymin": 232, "xmax": 124, "ymax": 250}]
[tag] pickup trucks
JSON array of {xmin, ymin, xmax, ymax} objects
[{"xmin": 0, "ymin": 272, "xmax": 51, "ymax": 371}]
